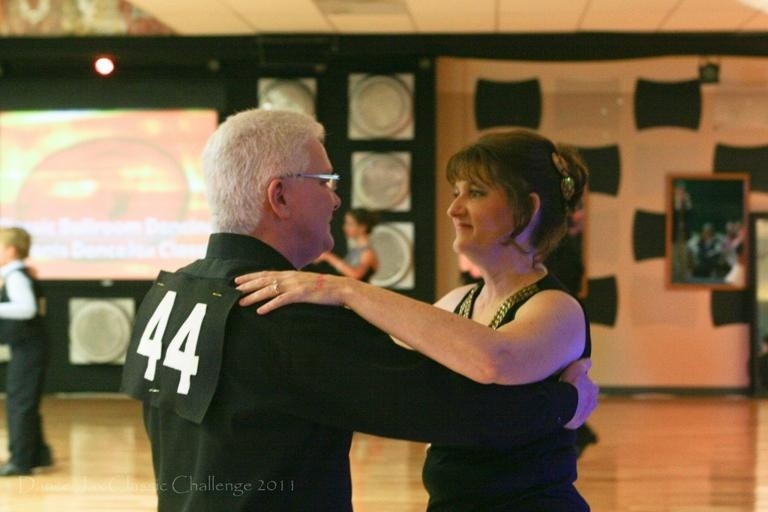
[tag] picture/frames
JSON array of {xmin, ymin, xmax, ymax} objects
[{"xmin": 666, "ymin": 170, "xmax": 749, "ymax": 291}]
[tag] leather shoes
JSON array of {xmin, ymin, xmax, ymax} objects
[{"xmin": 0, "ymin": 446, "xmax": 52, "ymax": 477}]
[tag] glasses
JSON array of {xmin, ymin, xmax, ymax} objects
[{"xmin": 278, "ymin": 170, "xmax": 341, "ymax": 192}]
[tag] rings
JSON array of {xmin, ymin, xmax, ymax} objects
[{"xmin": 271, "ymin": 279, "xmax": 281, "ymax": 294}]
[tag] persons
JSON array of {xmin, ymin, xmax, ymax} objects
[
  {"xmin": 313, "ymin": 206, "xmax": 379, "ymax": 285},
  {"xmin": 233, "ymin": 122, "xmax": 598, "ymax": 512},
  {"xmin": 0, "ymin": 223, "xmax": 52, "ymax": 476},
  {"xmin": 116, "ymin": 109, "xmax": 599, "ymax": 511},
  {"xmin": 686, "ymin": 217, "xmax": 748, "ymax": 286}
]
